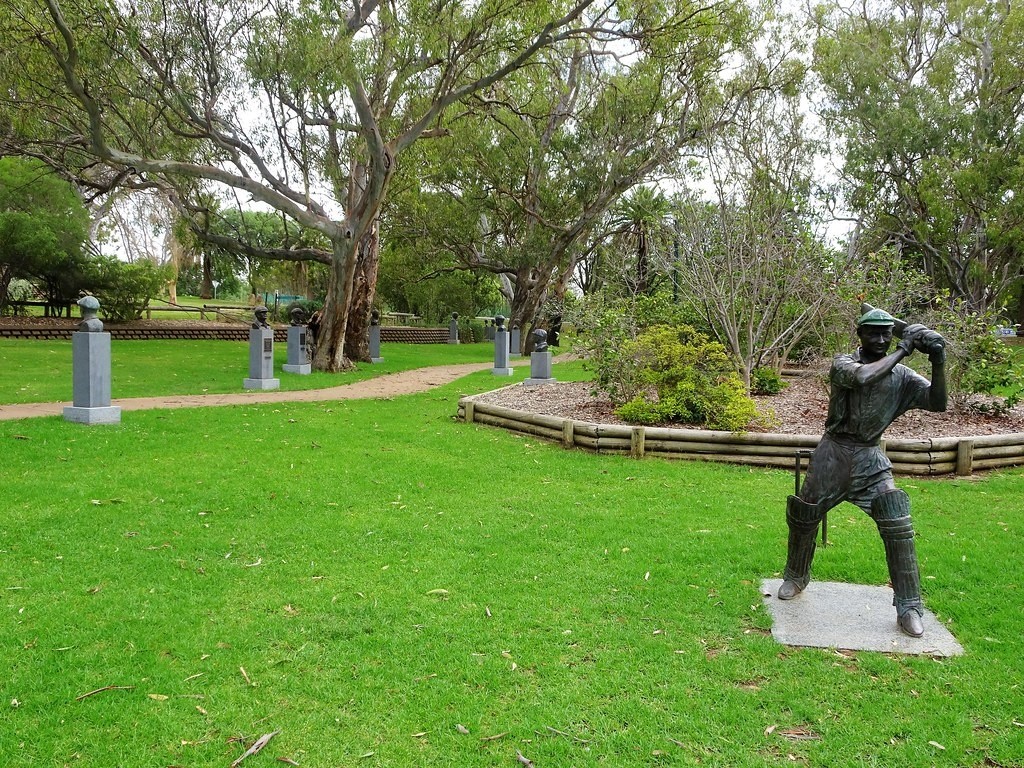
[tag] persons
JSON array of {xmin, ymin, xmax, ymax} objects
[
  {"xmin": 512, "ymin": 318, "xmax": 521, "ymax": 330},
  {"xmin": 76, "ymin": 296, "xmax": 105, "ymax": 332},
  {"xmin": 251, "ymin": 306, "xmax": 272, "ymax": 329},
  {"xmin": 778, "ymin": 309, "xmax": 947, "ymax": 638},
  {"xmin": 490, "ymin": 319, "xmax": 496, "ymax": 327},
  {"xmin": 495, "ymin": 314, "xmax": 507, "ymax": 332},
  {"xmin": 531, "ymin": 329, "xmax": 549, "ymax": 352},
  {"xmin": 290, "ymin": 308, "xmax": 305, "ymax": 327},
  {"xmin": 370, "ymin": 309, "xmax": 381, "ymax": 325},
  {"xmin": 451, "ymin": 312, "xmax": 458, "ymax": 319}
]
